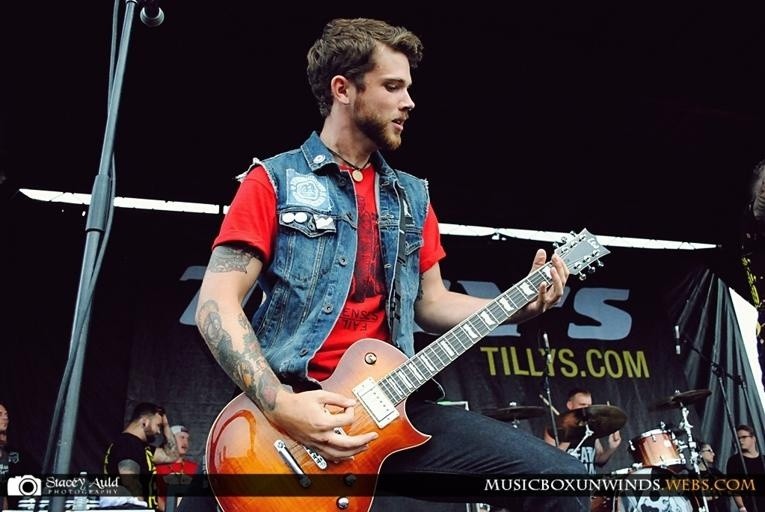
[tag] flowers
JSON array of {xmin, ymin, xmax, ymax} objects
[
  {"xmin": 674, "ymin": 326, "xmax": 681, "ymax": 355},
  {"xmin": 140, "ymin": 0, "xmax": 165, "ymax": 28},
  {"xmin": 543, "ymin": 334, "xmax": 551, "ymax": 364}
]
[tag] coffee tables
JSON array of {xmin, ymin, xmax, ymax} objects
[
  {"xmin": 479, "ymin": 406, "xmax": 544, "ymax": 422},
  {"xmin": 547, "ymin": 406, "xmax": 627, "ymax": 443},
  {"xmin": 649, "ymin": 389, "xmax": 712, "ymax": 410}
]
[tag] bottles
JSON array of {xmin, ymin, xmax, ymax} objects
[{"xmin": 159, "ymin": 425, "xmax": 189, "ymax": 448}]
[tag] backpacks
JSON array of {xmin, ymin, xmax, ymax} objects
[
  {"xmin": 591, "ymin": 466, "xmax": 700, "ymax": 512},
  {"xmin": 627, "ymin": 429, "xmax": 686, "ymax": 471}
]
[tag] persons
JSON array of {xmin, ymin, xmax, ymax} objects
[
  {"xmin": 724, "ymin": 423, "xmax": 765, "ymax": 511},
  {"xmin": 546, "ymin": 389, "xmax": 622, "ymax": 474},
  {"xmin": 175, "ymin": 14, "xmax": 595, "ymax": 512},
  {"xmin": 0, "ymin": 403, "xmax": 44, "ymax": 510},
  {"xmin": 101, "ymin": 401, "xmax": 180, "ymax": 509},
  {"xmin": 694, "ymin": 443, "xmax": 734, "ymax": 512},
  {"xmin": 156, "ymin": 422, "xmax": 200, "ymax": 512}
]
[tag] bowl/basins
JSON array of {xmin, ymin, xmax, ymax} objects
[{"xmin": 325, "ymin": 146, "xmax": 373, "ymax": 182}]
